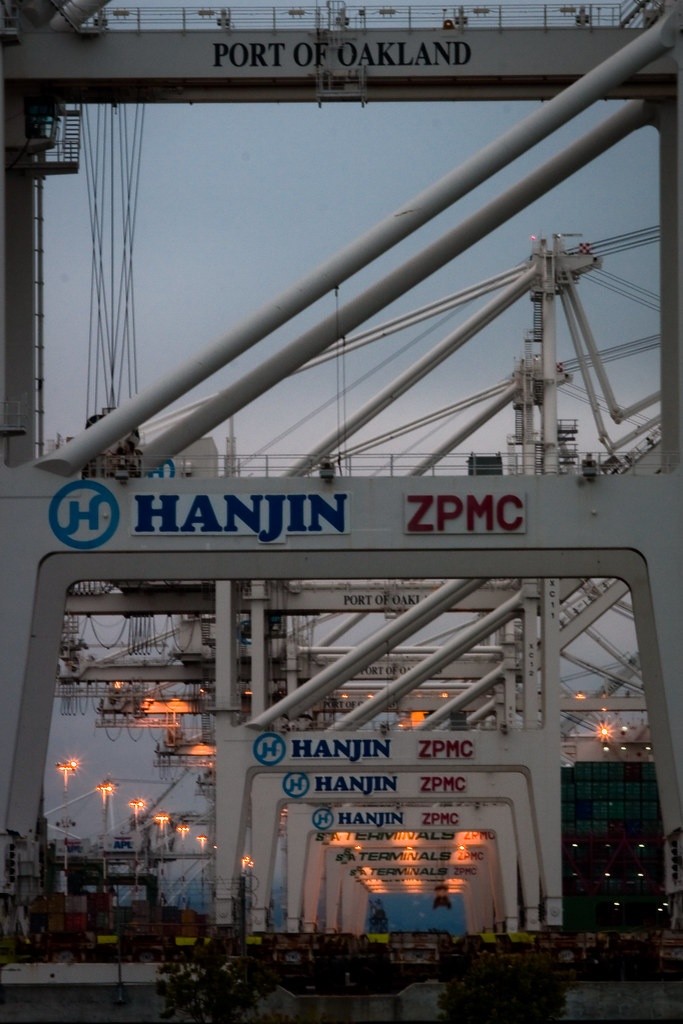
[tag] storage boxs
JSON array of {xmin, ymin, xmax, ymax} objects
[
  {"xmin": 562, "ymin": 761, "xmax": 665, "ymax": 896},
  {"xmin": 28, "ymin": 886, "xmax": 213, "ymax": 942}
]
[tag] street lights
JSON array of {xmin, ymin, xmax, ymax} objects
[
  {"xmin": 95, "ymin": 780, "xmax": 118, "ymax": 893},
  {"xmin": 54, "ymin": 759, "xmax": 78, "ymax": 897},
  {"xmin": 155, "ymin": 814, "xmax": 170, "ymax": 902},
  {"xmin": 176, "ymin": 824, "xmax": 191, "ymax": 909},
  {"xmin": 127, "ymin": 798, "xmax": 147, "ymax": 903},
  {"xmin": 196, "ymin": 834, "xmax": 208, "ymax": 913}
]
